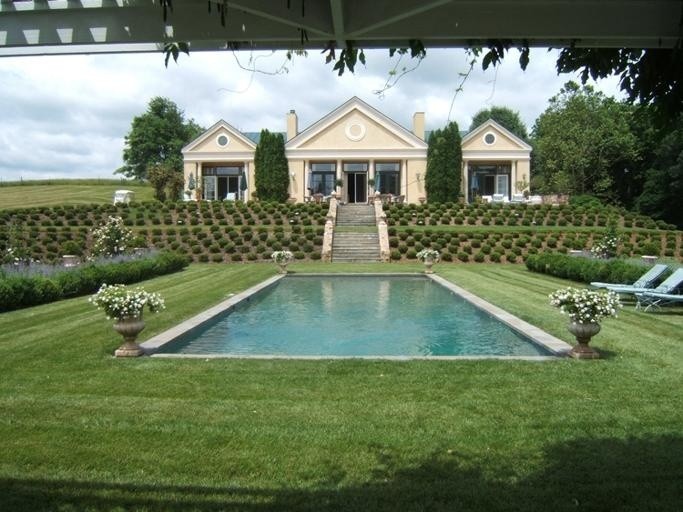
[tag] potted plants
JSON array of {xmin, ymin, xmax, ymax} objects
[
  {"xmin": 639, "ymin": 240, "xmax": 659, "ymax": 265},
  {"xmin": 368, "ymin": 179, "xmax": 375, "ymax": 197},
  {"xmin": 335, "ymin": 179, "xmax": 343, "ymax": 195}
]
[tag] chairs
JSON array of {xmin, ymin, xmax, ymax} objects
[{"xmin": 590, "ymin": 263, "xmax": 683, "ymax": 313}]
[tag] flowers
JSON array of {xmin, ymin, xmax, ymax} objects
[
  {"xmin": 416, "ymin": 248, "xmax": 440, "ymax": 265},
  {"xmin": 89, "ymin": 215, "xmax": 132, "ymax": 256},
  {"xmin": 549, "ymin": 285, "xmax": 624, "ymax": 325},
  {"xmin": 591, "ymin": 236, "xmax": 620, "ymax": 259},
  {"xmin": 88, "ymin": 283, "xmax": 166, "ymax": 321},
  {"xmin": 271, "ymin": 250, "xmax": 293, "ymax": 264}
]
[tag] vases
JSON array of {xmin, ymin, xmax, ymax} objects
[
  {"xmin": 112, "ymin": 320, "xmax": 146, "ymax": 358},
  {"xmin": 424, "ymin": 258, "xmax": 434, "ymax": 273},
  {"xmin": 276, "ymin": 260, "xmax": 289, "ymax": 273},
  {"xmin": 566, "ymin": 321, "xmax": 602, "ymax": 360}
]
